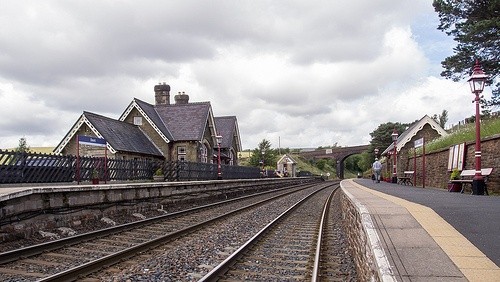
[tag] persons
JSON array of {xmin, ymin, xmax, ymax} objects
[{"xmin": 373, "ymin": 158, "xmax": 382, "ymax": 184}]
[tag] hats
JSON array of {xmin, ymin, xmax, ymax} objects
[{"xmin": 375, "ymin": 158, "xmax": 378, "ymax": 160}]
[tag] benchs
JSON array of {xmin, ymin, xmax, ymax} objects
[
  {"xmin": 448, "ymin": 167, "xmax": 493, "ymax": 194},
  {"xmin": 398, "ymin": 170, "xmax": 415, "ymax": 186}
]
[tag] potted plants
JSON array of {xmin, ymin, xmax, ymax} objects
[
  {"xmin": 91, "ymin": 168, "xmax": 100, "ymax": 185},
  {"xmin": 383, "ymin": 171, "xmax": 391, "ymax": 183},
  {"xmin": 152, "ymin": 168, "xmax": 165, "ymax": 182},
  {"xmin": 447, "ymin": 166, "xmax": 464, "ymax": 193}
]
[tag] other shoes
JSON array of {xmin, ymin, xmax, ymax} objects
[{"xmin": 376, "ymin": 180, "xmax": 380, "ymax": 183}]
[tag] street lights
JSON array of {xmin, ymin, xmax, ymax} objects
[
  {"xmin": 215, "ymin": 131, "xmax": 223, "ymax": 180},
  {"xmin": 467, "ymin": 59, "xmax": 489, "ymax": 196},
  {"xmin": 390, "ymin": 129, "xmax": 398, "ymax": 182}
]
